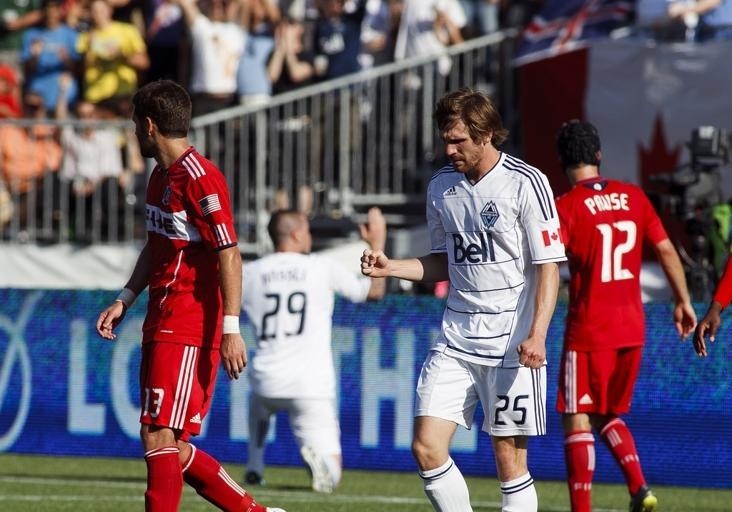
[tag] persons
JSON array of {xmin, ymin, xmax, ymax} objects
[
  {"xmin": 360, "ymin": 86, "xmax": 569, "ymax": 512},
  {"xmin": 96, "ymin": 82, "xmax": 286, "ymax": 512},
  {"xmin": 0, "ymin": 0, "xmax": 497, "ymax": 243},
  {"xmin": 241, "ymin": 205, "xmax": 387, "ymax": 493},
  {"xmin": 693, "ymin": 254, "xmax": 732, "ymax": 357},
  {"xmin": 637, "ymin": 1, "xmax": 732, "ymax": 43},
  {"xmin": 710, "ymin": 197, "xmax": 732, "ymax": 280},
  {"xmin": 553, "ymin": 118, "xmax": 698, "ymax": 512}
]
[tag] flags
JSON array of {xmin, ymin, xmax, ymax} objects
[{"xmin": 507, "ymin": 0, "xmax": 635, "ymax": 72}]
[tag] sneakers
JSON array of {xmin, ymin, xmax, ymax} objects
[
  {"xmin": 630, "ymin": 487, "xmax": 658, "ymax": 512},
  {"xmin": 245, "ymin": 470, "xmax": 262, "ymax": 484},
  {"xmin": 299, "ymin": 445, "xmax": 335, "ymax": 494}
]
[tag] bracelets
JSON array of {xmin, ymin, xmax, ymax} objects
[
  {"xmin": 115, "ymin": 288, "xmax": 136, "ymax": 310},
  {"xmin": 222, "ymin": 315, "xmax": 241, "ymax": 334}
]
